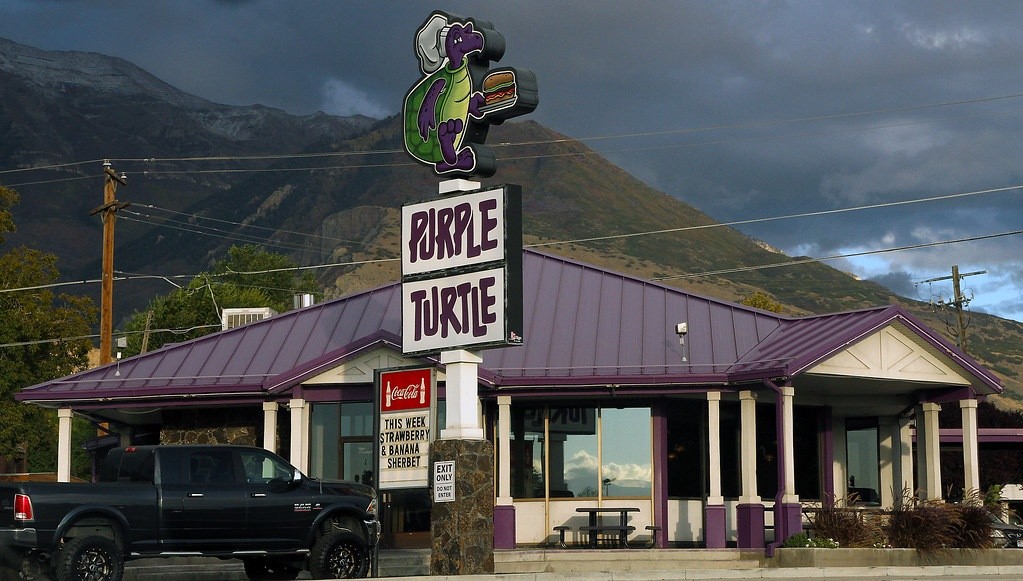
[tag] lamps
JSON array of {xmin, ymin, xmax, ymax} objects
[
  {"xmin": 113, "ymin": 336, "xmax": 127, "ymax": 376},
  {"xmin": 675, "ymin": 321, "xmax": 689, "ymax": 362}
]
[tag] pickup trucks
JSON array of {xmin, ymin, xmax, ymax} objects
[{"xmin": 0, "ymin": 444, "xmax": 382, "ymax": 581}]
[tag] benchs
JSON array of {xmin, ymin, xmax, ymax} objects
[
  {"xmin": 553, "ymin": 526, "xmax": 571, "ymax": 549},
  {"xmin": 645, "ymin": 525, "xmax": 660, "ymax": 548},
  {"xmin": 579, "ymin": 526, "xmax": 636, "ymax": 549}
]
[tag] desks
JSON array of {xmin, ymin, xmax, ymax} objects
[{"xmin": 576, "ymin": 508, "xmax": 640, "ymax": 549}]
